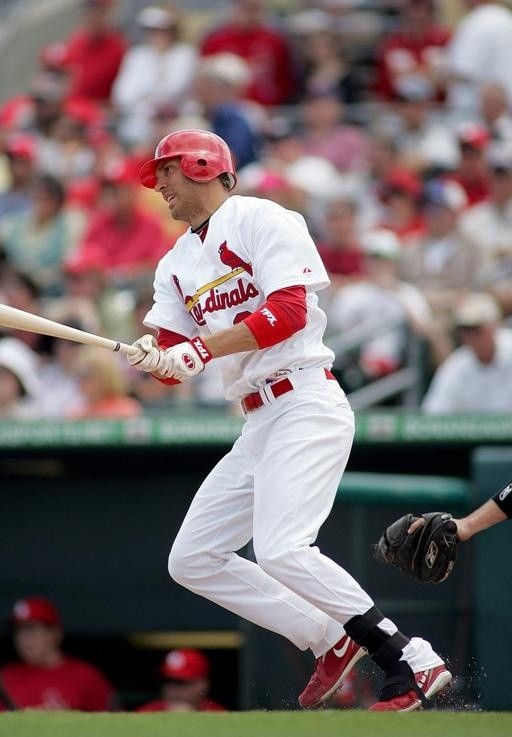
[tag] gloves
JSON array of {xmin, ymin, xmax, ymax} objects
[
  {"xmin": 158, "ymin": 335, "xmax": 213, "ymax": 383},
  {"xmin": 126, "ymin": 333, "xmax": 167, "ymax": 373}
]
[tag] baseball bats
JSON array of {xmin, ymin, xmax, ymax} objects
[{"xmin": 1, "ymin": 303, "xmax": 141, "ymax": 357}]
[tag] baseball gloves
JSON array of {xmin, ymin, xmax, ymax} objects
[{"xmin": 373, "ymin": 512, "xmax": 462, "ymax": 584}]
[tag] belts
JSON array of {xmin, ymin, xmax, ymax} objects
[{"xmin": 241, "ymin": 368, "xmax": 338, "ymax": 414}]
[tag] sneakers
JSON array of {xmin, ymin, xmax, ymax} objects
[
  {"xmin": 368, "ymin": 663, "xmax": 453, "ymax": 713},
  {"xmin": 299, "ymin": 632, "xmax": 368, "ymax": 708}
]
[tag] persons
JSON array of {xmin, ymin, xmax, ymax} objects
[
  {"xmin": 127, "ymin": 127, "xmax": 453, "ymax": 710},
  {"xmin": 1, "ymin": 594, "xmax": 123, "ymax": 711},
  {"xmin": 2, "ymin": 1, "xmax": 512, "ymax": 419},
  {"xmin": 375, "ymin": 479, "xmax": 510, "ymax": 581},
  {"xmin": 134, "ymin": 644, "xmax": 228, "ymax": 712}
]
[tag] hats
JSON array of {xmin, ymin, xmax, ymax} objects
[
  {"xmin": 160, "ymin": 646, "xmax": 210, "ymax": 683},
  {"xmin": 5, "ymin": 596, "xmax": 62, "ymax": 627}
]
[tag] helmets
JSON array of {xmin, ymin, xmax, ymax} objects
[{"xmin": 139, "ymin": 128, "xmax": 237, "ymax": 192}]
